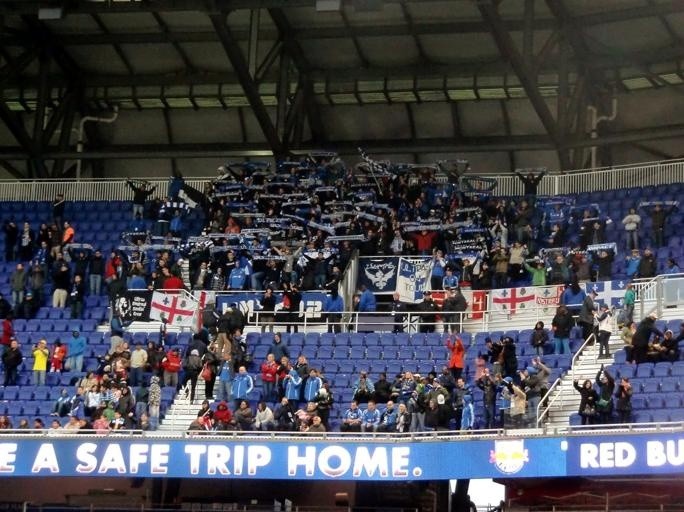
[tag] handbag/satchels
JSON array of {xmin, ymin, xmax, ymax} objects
[
  {"xmin": 596, "ymin": 397, "xmax": 612, "ymax": 409},
  {"xmin": 201, "ymin": 361, "xmax": 212, "ymax": 382},
  {"xmin": 580, "ymin": 404, "xmax": 597, "ymax": 416}
]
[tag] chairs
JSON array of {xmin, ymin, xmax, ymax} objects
[{"xmin": 1, "ymin": 181, "xmax": 684, "ymax": 436}]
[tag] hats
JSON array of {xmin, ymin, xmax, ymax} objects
[
  {"xmin": 428, "ymin": 372, "xmax": 437, "ymax": 378},
  {"xmin": 433, "ymin": 378, "xmax": 439, "ymax": 383},
  {"xmin": 665, "ymin": 328, "xmax": 673, "ymax": 340},
  {"xmin": 39, "ymin": 340, "xmax": 46, "ymax": 344},
  {"xmin": 413, "ymin": 374, "xmax": 421, "ymax": 378},
  {"xmin": 647, "ymin": 311, "xmax": 657, "ymax": 321}
]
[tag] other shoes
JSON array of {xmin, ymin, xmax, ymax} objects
[
  {"xmin": 186, "ymin": 391, "xmax": 189, "ymax": 398},
  {"xmin": 190, "ymin": 400, "xmax": 193, "ymax": 405},
  {"xmin": 206, "ymin": 397, "xmax": 214, "ymax": 399}
]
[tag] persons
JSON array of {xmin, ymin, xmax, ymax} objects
[
  {"xmin": 51, "ymin": 340, "xmax": 182, "ymax": 434},
  {"xmin": 447, "ymin": 336, "xmax": 464, "ymax": 379},
  {"xmin": 529, "ymin": 321, "xmax": 549, "ymax": 355},
  {"xmin": 184, "ymin": 328, "xmax": 334, "ymax": 435},
  {"xmin": 541, "ymin": 200, "xmax": 680, "ymax": 285},
  {"xmin": 105, "ymin": 167, "xmax": 548, "ymax": 334},
  {"xmin": 551, "ymin": 281, "xmax": 598, "ymax": 354},
  {"xmin": 617, "ymin": 283, "xmax": 681, "ymax": 364},
  {"xmin": 0, "ymin": 315, "xmax": 86, "ymax": 387},
  {"xmin": 474, "ymin": 336, "xmax": 551, "ymax": 432},
  {"xmin": 340, "ymin": 364, "xmax": 474, "ymax": 435},
  {"xmin": 0, "ymin": 415, "xmax": 44, "ymax": 434},
  {"xmin": 0, "ymin": 194, "xmax": 103, "ymax": 318},
  {"xmin": 574, "ymin": 363, "xmax": 633, "ymax": 430},
  {"xmin": 597, "ymin": 304, "xmax": 611, "ymax": 358}
]
